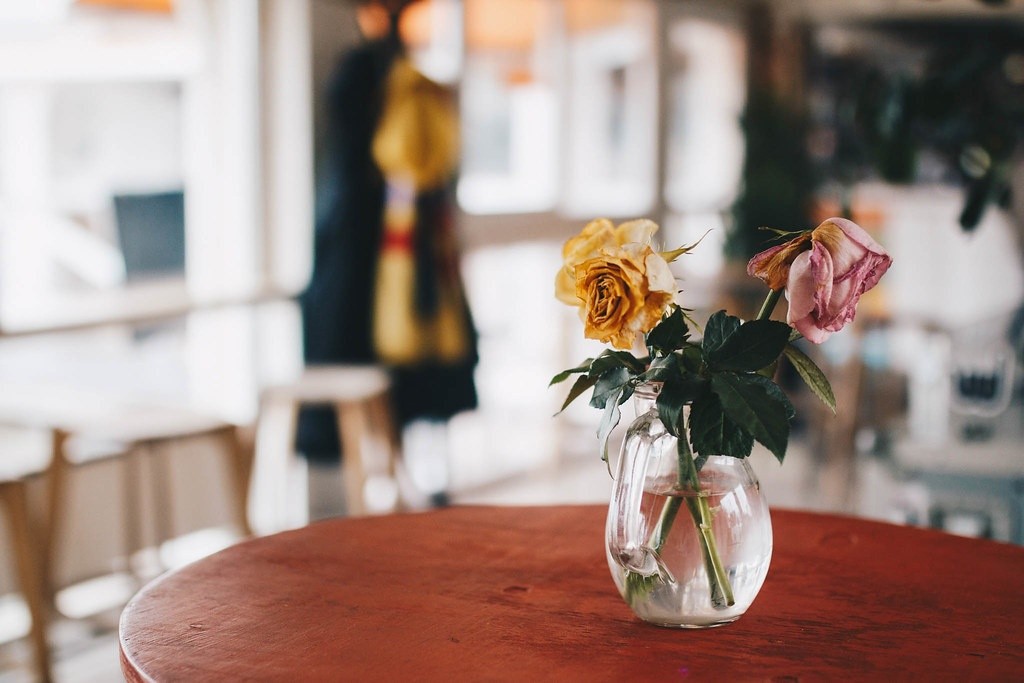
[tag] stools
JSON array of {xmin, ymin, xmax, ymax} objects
[{"xmin": 262, "ymin": 363, "xmax": 407, "ymax": 522}]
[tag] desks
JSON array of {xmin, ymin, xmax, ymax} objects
[{"xmin": 115, "ymin": 504, "xmax": 1024, "ymax": 683}]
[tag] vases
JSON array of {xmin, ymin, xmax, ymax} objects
[{"xmin": 604, "ymin": 381, "xmax": 772, "ymax": 628}]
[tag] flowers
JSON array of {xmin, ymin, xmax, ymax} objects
[{"xmin": 552, "ymin": 216, "xmax": 893, "ymax": 608}]
